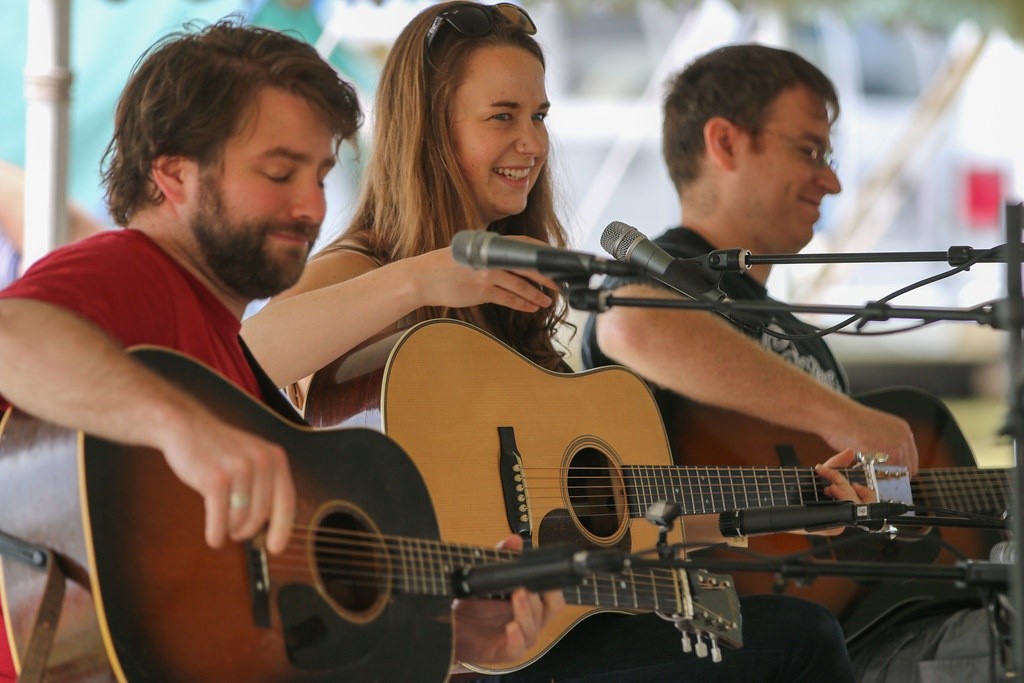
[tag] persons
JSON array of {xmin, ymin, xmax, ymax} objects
[{"xmin": 0, "ymin": 0, "xmax": 1024, "ymax": 683}]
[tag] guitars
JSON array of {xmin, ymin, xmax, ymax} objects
[
  {"xmin": 0, "ymin": 340, "xmax": 749, "ymax": 683},
  {"xmin": 301, "ymin": 314, "xmax": 920, "ymax": 678},
  {"xmin": 650, "ymin": 382, "xmax": 1024, "ymax": 623}
]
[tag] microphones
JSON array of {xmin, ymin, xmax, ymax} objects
[
  {"xmin": 600, "ymin": 224, "xmax": 768, "ymax": 336},
  {"xmin": 451, "ymin": 229, "xmax": 638, "ymax": 279},
  {"xmin": 721, "ymin": 502, "xmax": 908, "ymax": 538},
  {"xmin": 450, "ymin": 550, "xmax": 637, "ymax": 599}
]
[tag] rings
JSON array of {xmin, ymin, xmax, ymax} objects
[{"xmin": 228, "ymin": 492, "xmax": 250, "ymax": 507}]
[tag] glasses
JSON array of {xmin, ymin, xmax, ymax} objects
[
  {"xmin": 426, "ymin": 2, "xmax": 540, "ymax": 38},
  {"xmin": 764, "ymin": 127, "xmax": 839, "ymax": 173}
]
